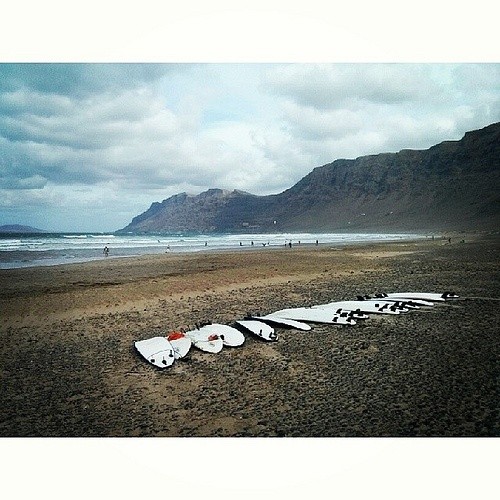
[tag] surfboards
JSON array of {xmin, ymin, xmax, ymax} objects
[{"xmin": 135, "ymin": 291, "xmax": 460, "ymax": 368}]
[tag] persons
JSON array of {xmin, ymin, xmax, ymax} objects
[{"xmin": 103, "ymin": 246, "xmax": 108, "ymax": 255}]
